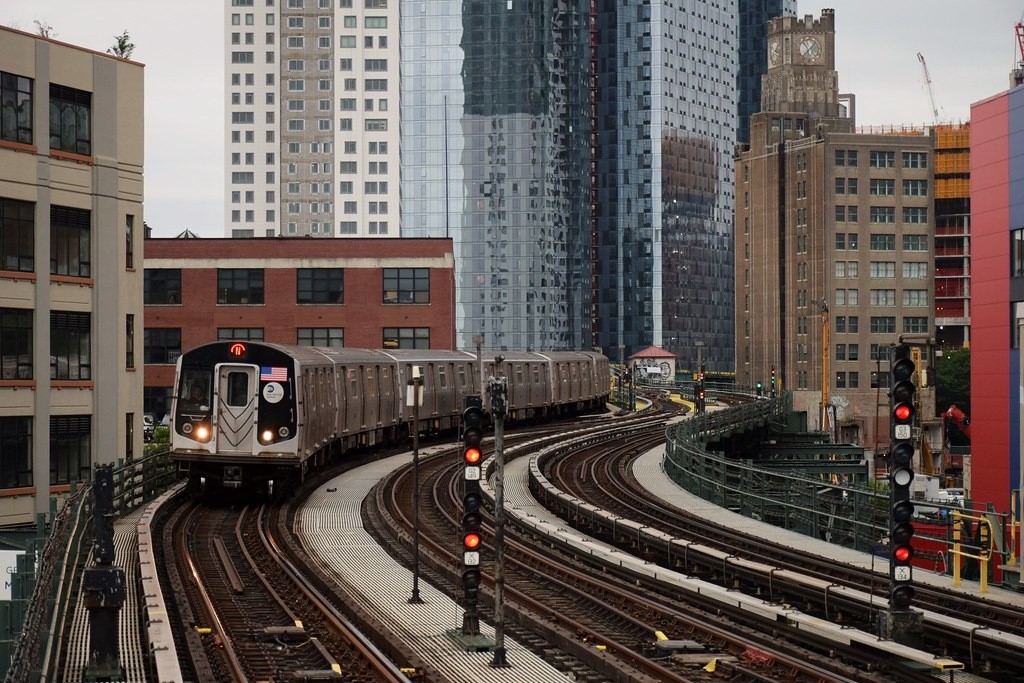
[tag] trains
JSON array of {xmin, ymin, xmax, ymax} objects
[{"xmin": 168, "ymin": 338, "xmax": 611, "ymax": 501}]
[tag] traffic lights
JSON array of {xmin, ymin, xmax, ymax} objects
[
  {"xmin": 771, "ymin": 365, "xmax": 775, "ymax": 398},
  {"xmin": 446, "ymin": 394, "xmax": 494, "ymax": 650},
  {"xmin": 877, "ymin": 344, "xmax": 924, "ymax": 641},
  {"xmin": 756, "ymin": 380, "xmax": 761, "ymax": 396},
  {"xmin": 699, "ymin": 362, "xmax": 705, "ymax": 413}
]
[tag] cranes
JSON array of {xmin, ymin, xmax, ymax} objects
[
  {"xmin": 917, "ymin": 52, "xmax": 940, "ymax": 126},
  {"xmin": 1014, "ymin": 23, "xmax": 1024, "ymax": 86}
]
